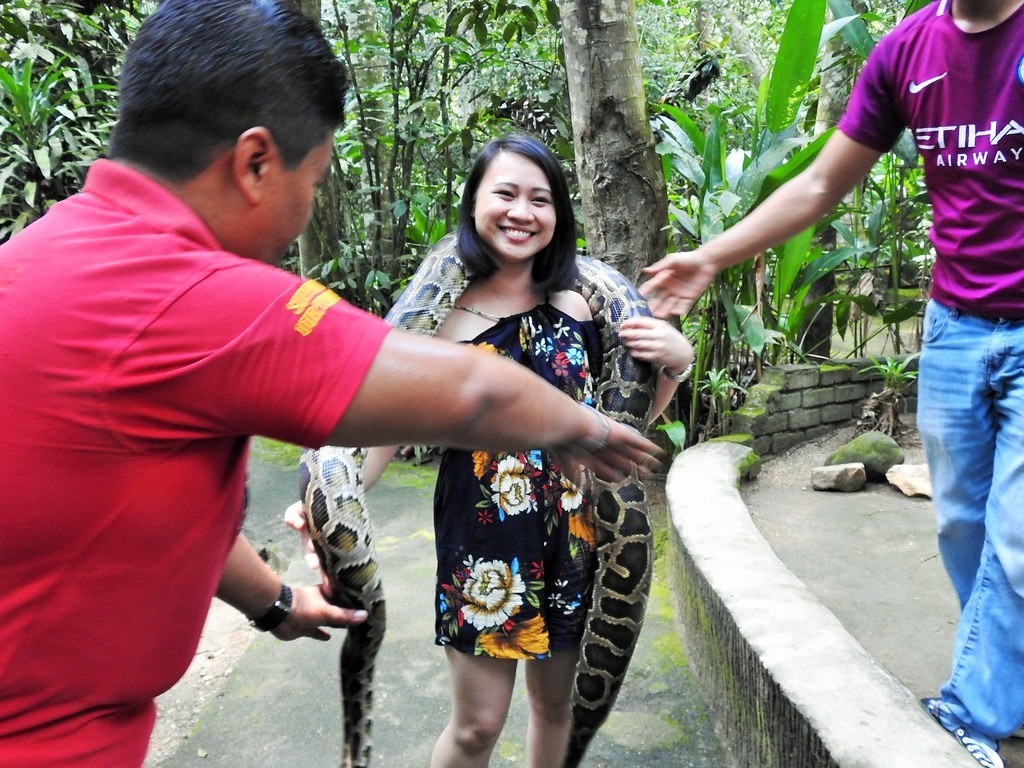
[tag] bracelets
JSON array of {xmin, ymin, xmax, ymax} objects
[
  {"xmin": 250, "ymin": 584, "xmax": 293, "ymax": 632},
  {"xmin": 659, "ymin": 363, "xmax": 694, "ymax": 382},
  {"xmin": 578, "ymin": 400, "xmax": 611, "ymax": 455}
]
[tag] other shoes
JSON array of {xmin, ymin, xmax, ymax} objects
[
  {"xmin": 1012, "ymin": 724, "xmax": 1024, "ymax": 737},
  {"xmin": 924, "ymin": 696, "xmax": 1006, "ymax": 768}
]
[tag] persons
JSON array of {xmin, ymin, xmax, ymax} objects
[
  {"xmin": 280, "ymin": 132, "xmax": 696, "ymax": 768},
  {"xmin": 634, "ymin": 0, "xmax": 1024, "ymax": 768},
  {"xmin": 0, "ymin": 1, "xmax": 665, "ymax": 768}
]
